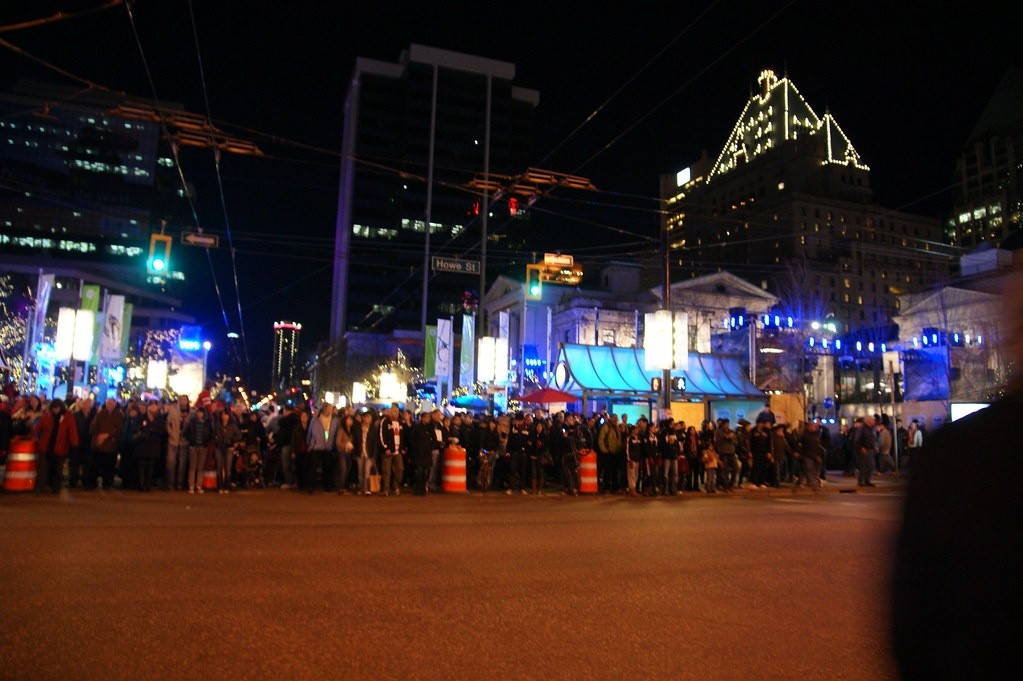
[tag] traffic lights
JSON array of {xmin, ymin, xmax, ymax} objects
[
  {"xmin": 650, "ymin": 377, "xmax": 662, "ymax": 392},
  {"xmin": 526, "ymin": 265, "xmax": 542, "ymax": 301},
  {"xmin": 672, "ymin": 377, "xmax": 687, "ymax": 392},
  {"xmin": 147, "ymin": 233, "xmax": 172, "ymax": 276}
]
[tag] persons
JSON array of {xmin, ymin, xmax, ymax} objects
[
  {"xmin": 880, "ymin": 224, "xmax": 1023, "ymax": 681},
  {"xmin": 1, "ymin": 376, "xmax": 925, "ymax": 501}
]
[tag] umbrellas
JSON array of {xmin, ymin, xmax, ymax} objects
[
  {"xmin": 514, "ymin": 387, "xmax": 579, "ymax": 417},
  {"xmin": 449, "ymin": 392, "xmax": 501, "ymax": 415}
]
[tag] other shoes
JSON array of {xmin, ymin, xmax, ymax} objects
[
  {"xmin": 188, "ymin": 487, "xmax": 194, "ymax": 494},
  {"xmin": 196, "ymin": 487, "xmax": 204, "ymax": 494}
]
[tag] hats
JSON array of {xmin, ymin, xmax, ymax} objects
[{"xmin": 513, "ymin": 411, "xmax": 524, "ymax": 420}]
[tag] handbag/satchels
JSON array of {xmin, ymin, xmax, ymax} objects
[{"xmin": 369, "ymin": 475, "xmax": 381, "ymax": 492}]
